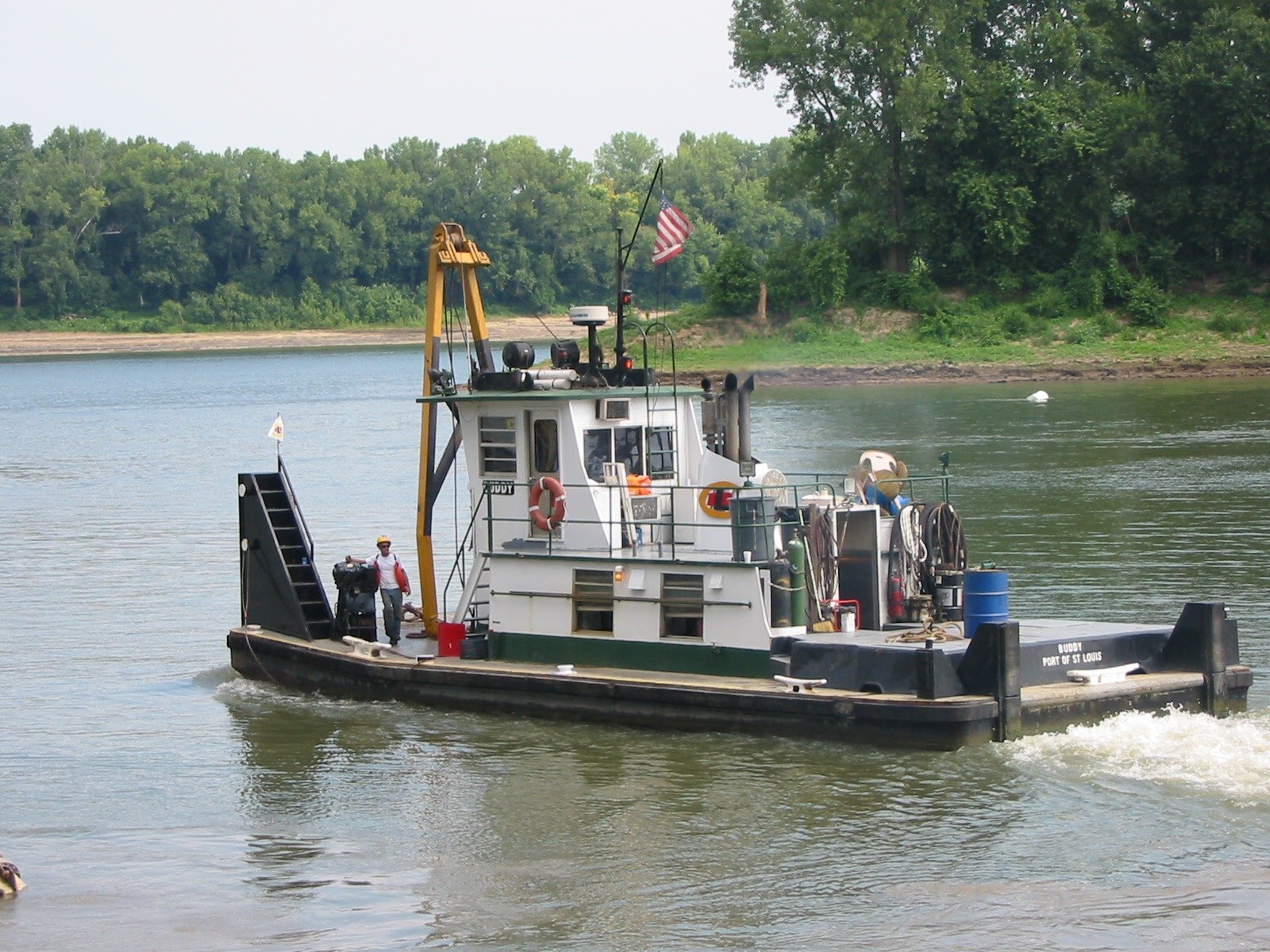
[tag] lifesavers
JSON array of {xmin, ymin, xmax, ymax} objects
[{"xmin": 528, "ymin": 476, "xmax": 566, "ymax": 532}]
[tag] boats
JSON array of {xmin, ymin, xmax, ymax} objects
[{"xmin": 227, "ymin": 222, "xmax": 1255, "ymax": 755}]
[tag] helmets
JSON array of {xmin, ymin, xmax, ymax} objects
[{"xmin": 377, "ymin": 534, "xmax": 391, "ymax": 546}]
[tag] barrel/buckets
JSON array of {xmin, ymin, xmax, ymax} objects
[
  {"xmin": 962, "ymin": 568, "xmax": 1009, "ymax": 638},
  {"xmin": 728, "ymin": 496, "xmax": 778, "ymax": 560}
]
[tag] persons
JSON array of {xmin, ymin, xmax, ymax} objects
[
  {"xmin": 587, "ymin": 438, "xmax": 607, "ymax": 480},
  {"xmin": 346, "ymin": 535, "xmax": 411, "ymax": 645}
]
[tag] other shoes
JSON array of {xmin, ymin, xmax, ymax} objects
[
  {"xmin": 390, "ymin": 637, "xmax": 398, "ymax": 645},
  {"xmin": 392, "ymin": 634, "xmax": 402, "ymax": 640}
]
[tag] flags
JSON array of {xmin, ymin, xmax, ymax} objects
[
  {"xmin": 652, "ymin": 193, "xmax": 695, "ymax": 265},
  {"xmin": 268, "ymin": 417, "xmax": 283, "ymax": 441}
]
